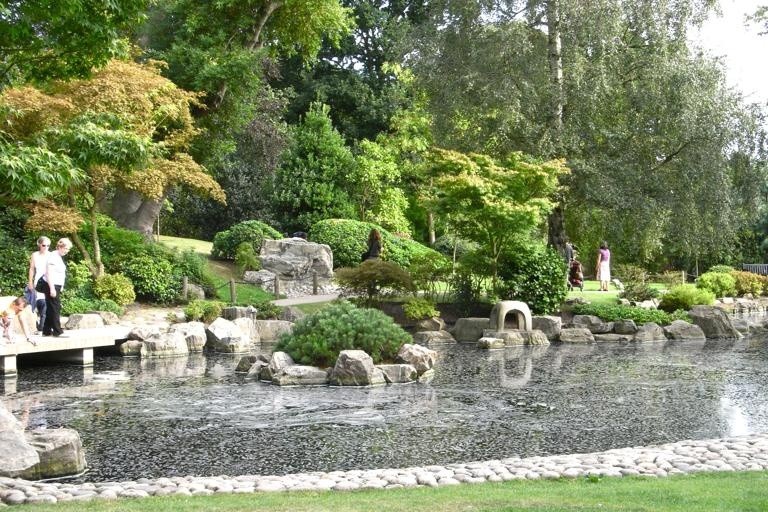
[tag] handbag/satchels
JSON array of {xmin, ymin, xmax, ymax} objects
[{"xmin": 36, "ymin": 275, "xmax": 55, "ymax": 295}]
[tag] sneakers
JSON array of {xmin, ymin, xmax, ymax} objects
[{"xmin": 41, "ymin": 333, "xmax": 71, "ymax": 339}]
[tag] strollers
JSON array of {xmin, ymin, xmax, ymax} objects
[{"xmin": 569, "ymin": 261, "xmax": 584, "ymax": 293}]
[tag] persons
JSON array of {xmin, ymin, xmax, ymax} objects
[
  {"xmin": 41, "ymin": 236, "xmax": 73, "ymax": 337},
  {"xmin": 595, "ymin": 239, "xmax": 611, "ymax": 294},
  {"xmin": 26, "ymin": 237, "xmax": 52, "ymax": 330},
  {"xmin": 365, "ymin": 230, "xmax": 384, "ymax": 295},
  {"xmin": 1, "ymin": 294, "xmax": 38, "ymax": 347},
  {"xmin": 561, "ymin": 239, "xmax": 576, "ymax": 291}
]
